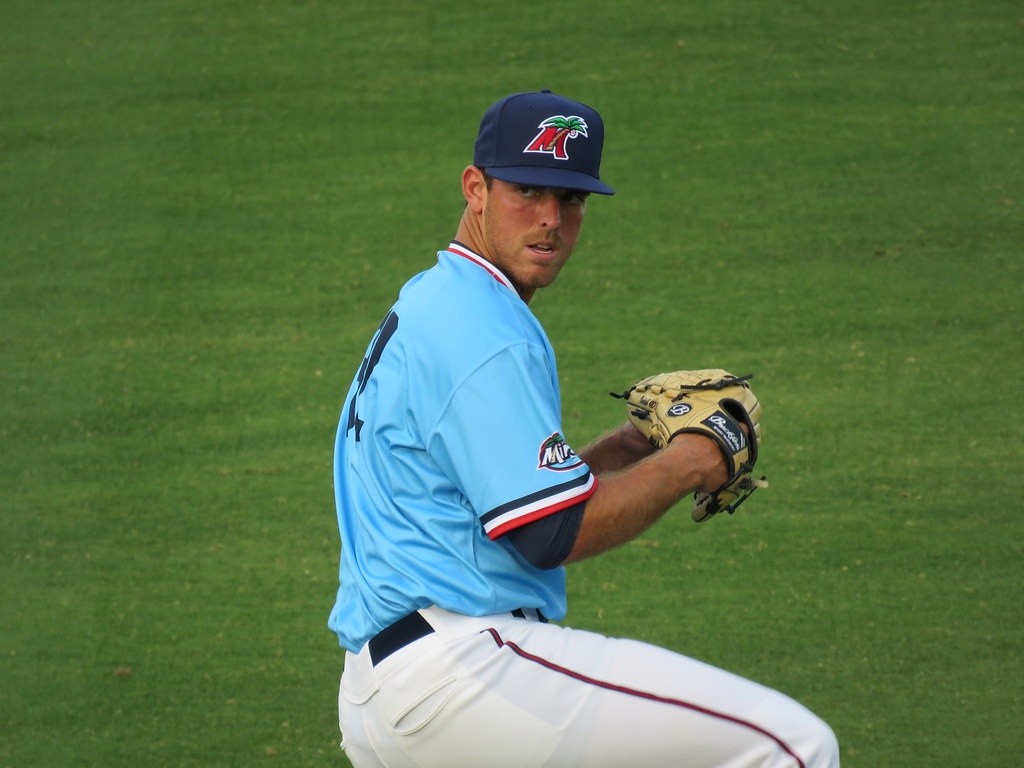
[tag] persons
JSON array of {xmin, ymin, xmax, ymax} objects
[{"xmin": 326, "ymin": 90, "xmax": 842, "ymax": 768}]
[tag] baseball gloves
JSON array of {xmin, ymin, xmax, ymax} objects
[{"xmin": 607, "ymin": 367, "xmax": 769, "ymax": 525}]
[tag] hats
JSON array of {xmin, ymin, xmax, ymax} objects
[{"xmin": 473, "ymin": 88, "xmax": 617, "ymax": 195}]
